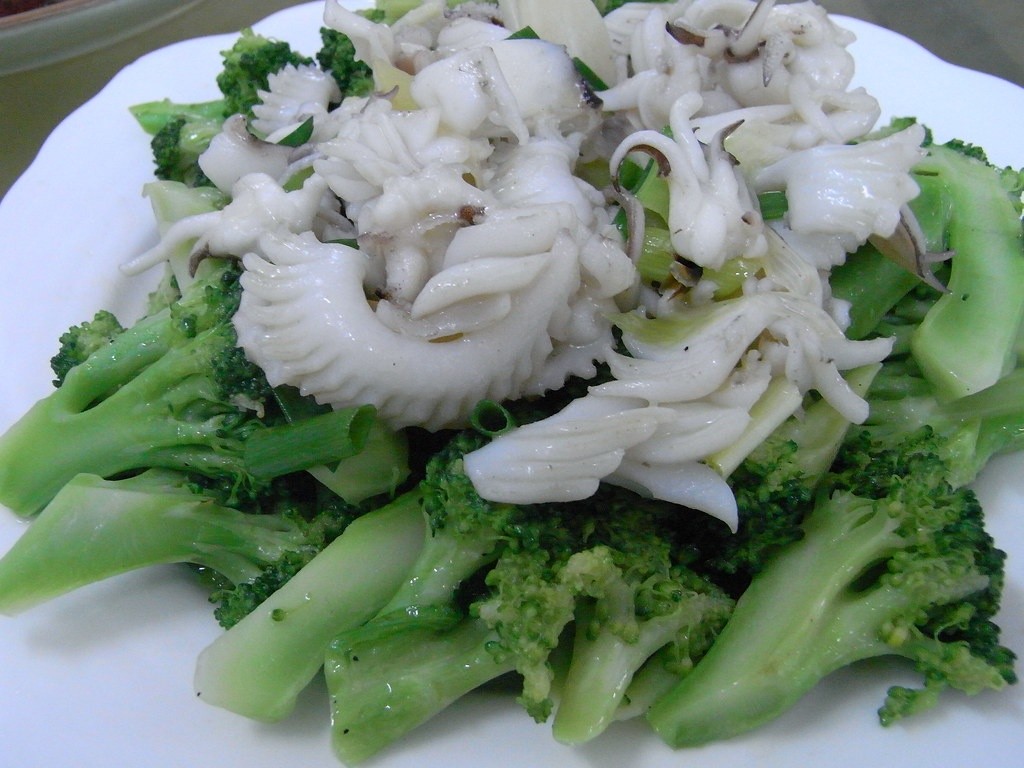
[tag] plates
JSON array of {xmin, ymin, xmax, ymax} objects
[{"xmin": 0, "ymin": 0, "xmax": 1024, "ymax": 768}]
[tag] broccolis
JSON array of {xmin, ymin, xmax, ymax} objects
[{"xmin": 0, "ymin": 0, "xmax": 1024, "ymax": 759}]
